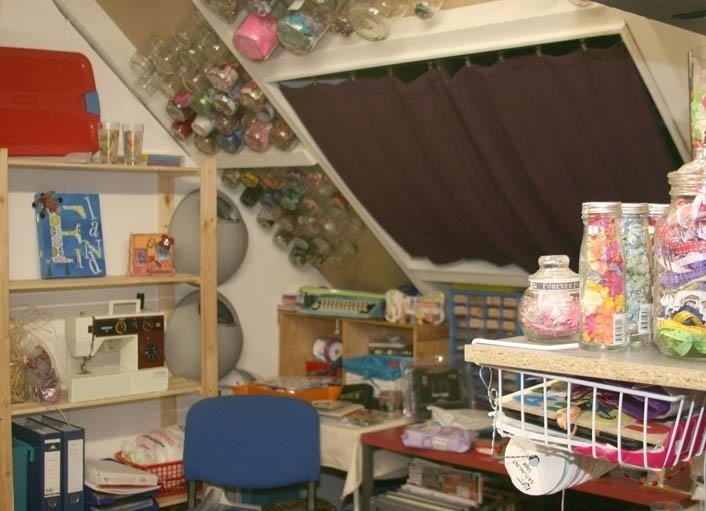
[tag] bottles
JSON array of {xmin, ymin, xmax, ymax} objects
[
  {"xmin": 621, "ymin": 203, "xmax": 671, "ymax": 351},
  {"xmin": 578, "ymin": 200, "xmax": 630, "ymax": 355},
  {"xmin": 201, "ymin": 2, "xmax": 444, "ymax": 61},
  {"xmin": 219, "ymin": 167, "xmax": 366, "ymax": 269},
  {"xmin": 130, "ymin": 11, "xmax": 301, "ymax": 155},
  {"xmin": 653, "ymin": 168, "xmax": 706, "ymax": 364},
  {"xmin": 516, "ymin": 253, "xmax": 581, "ymax": 346}
]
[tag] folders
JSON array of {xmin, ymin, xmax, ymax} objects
[{"xmin": 12, "ymin": 414, "xmax": 85, "ymax": 511}]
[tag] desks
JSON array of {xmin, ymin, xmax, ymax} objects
[
  {"xmin": 316, "ymin": 411, "xmax": 411, "ymax": 511},
  {"xmin": 360, "ymin": 422, "xmax": 696, "ymax": 511}
]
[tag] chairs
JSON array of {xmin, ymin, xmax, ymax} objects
[{"xmin": 183, "ymin": 394, "xmax": 320, "ymax": 511}]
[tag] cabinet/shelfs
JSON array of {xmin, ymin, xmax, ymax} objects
[
  {"xmin": 279, "ymin": 309, "xmax": 448, "ymax": 408},
  {"xmin": 0, "ymin": 147, "xmax": 218, "ymax": 511}
]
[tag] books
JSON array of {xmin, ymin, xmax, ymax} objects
[
  {"xmin": 386, "ymin": 455, "xmax": 484, "ymax": 511},
  {"xmin": 32, "ymin": 190, "xmax": 107, "ymax": 279},
  {"xmin": 315, "ymin": 400, "xmax": 365, "ymax": 419},
  {"xmin": 83, "ymin": 459, "xmax": 160, "ymax": 510}
]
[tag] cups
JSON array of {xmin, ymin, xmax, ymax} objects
[
  {"xmin": 96, "ymin": 121, "xmax": 121, "ymax": 165},
  {"xmin": 121, "ymin": 124, "xmax": 145, "ymax": 166}
]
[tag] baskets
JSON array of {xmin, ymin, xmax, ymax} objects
[
  {"xmin": 476, "ymin": 362, "xmax": 706, "ymax": 471},
  {"xmin": 115, "ymin": 451, "xmax": 202, "ymax": 498}
]
